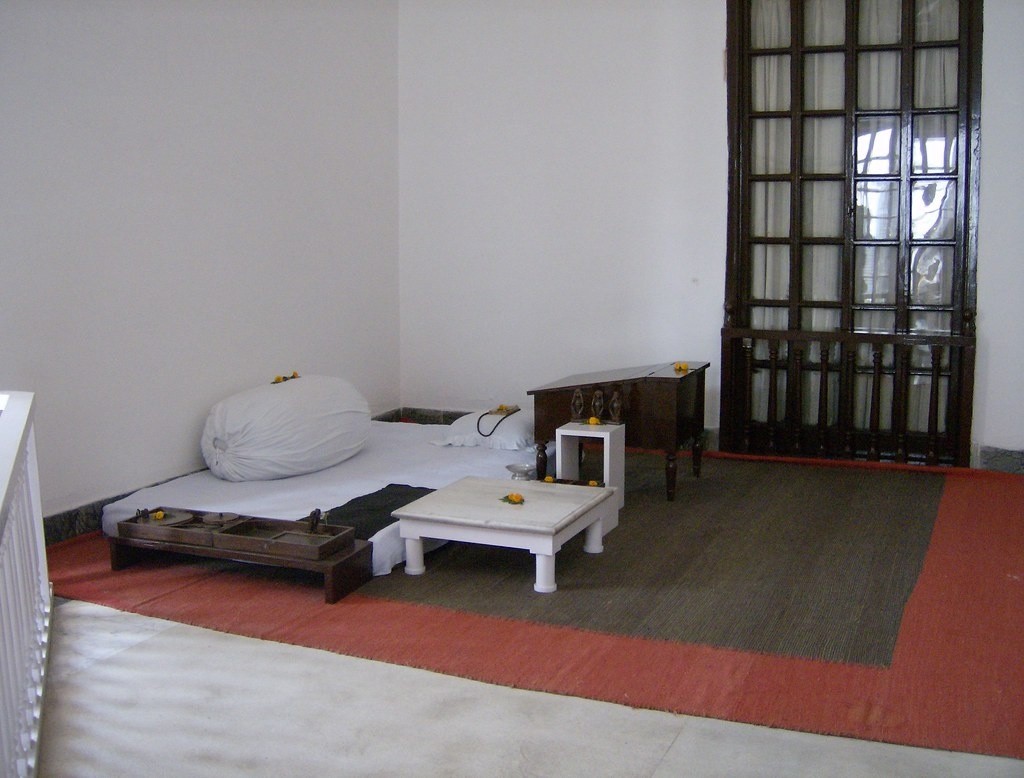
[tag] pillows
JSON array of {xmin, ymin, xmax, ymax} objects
[{"xmin": 428, "ymin": 410, "xmax": 534, "ymax": 450}]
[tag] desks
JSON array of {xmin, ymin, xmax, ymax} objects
[{"xmin": 391, "ymin": 476, "xmax": 614, "ymax": 593}]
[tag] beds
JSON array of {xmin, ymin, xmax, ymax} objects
[{"xmin": 101, "ymin": 419, "xmax": 556, "ymax": 603}]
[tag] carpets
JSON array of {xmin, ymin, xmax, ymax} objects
[{"xmin": 147, "ymin": 448, "xmax": 947, "ymax": 669}]
